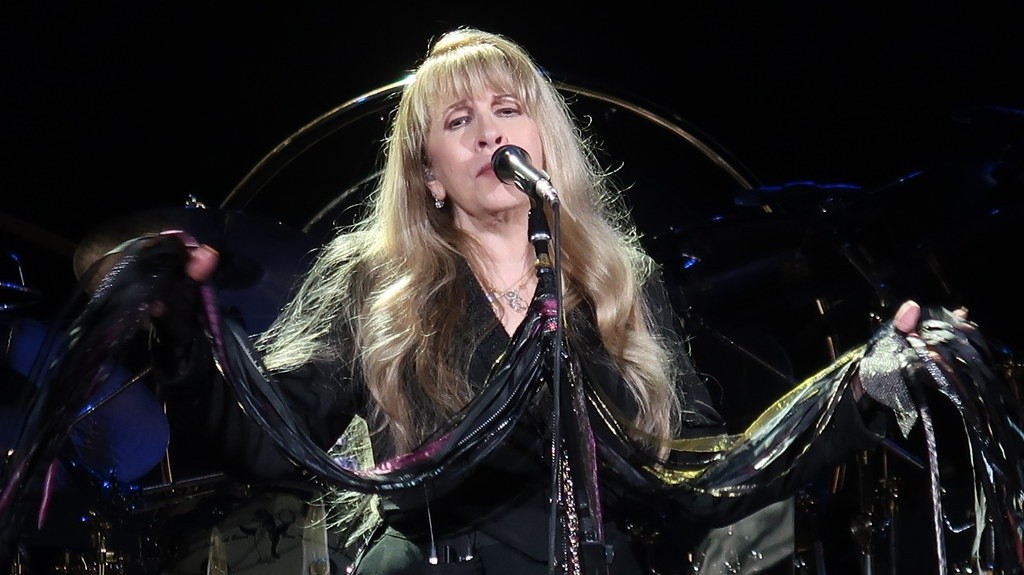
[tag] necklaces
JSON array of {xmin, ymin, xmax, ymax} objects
[{"xmin": 486, "ymin": 264, "xmax": 536, "ymax": 314}]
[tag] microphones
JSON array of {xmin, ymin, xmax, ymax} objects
[{"xmin": 490, "ymin": 143, "xmax": 559, "ymax": 208}]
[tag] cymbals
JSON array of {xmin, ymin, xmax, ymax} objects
[{"xmin": 70, "ymin": 192, "xmax": 293, "ymax": 303}]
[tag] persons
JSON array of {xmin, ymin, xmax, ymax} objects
[
  {"xmin": 147, "ymin": 29, "xmax": 974, "ymax": 575},
  {"xmin": 72, "ymin": 225, "xmax": 330, "ymax": 575}
]
